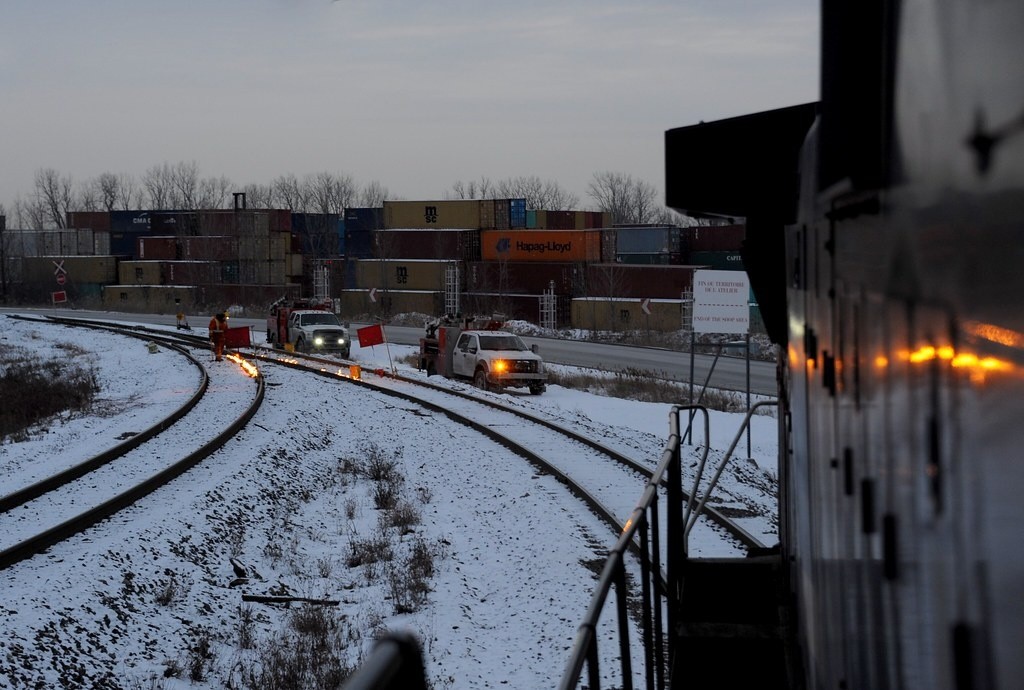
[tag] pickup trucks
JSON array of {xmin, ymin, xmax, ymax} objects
[
  {"xmin": 267, "ymin": 309, "xmax": 351, "ymax": 359},
  {"xmin": 418, "ymin": 328, "xmax": 548, "ymax": 395}
]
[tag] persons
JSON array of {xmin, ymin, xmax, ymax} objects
[{"xmin": 208, "ymin": 312, "xmax": 229, "ymax": 361}]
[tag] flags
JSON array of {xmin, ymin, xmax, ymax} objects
[
  {"xmin": 224, "ymin": 326, "xmax": 252, "ymax": 349},
  {"xmin": 356, "ymin": 325, "xmax": 384, "ymax": 348}
]
